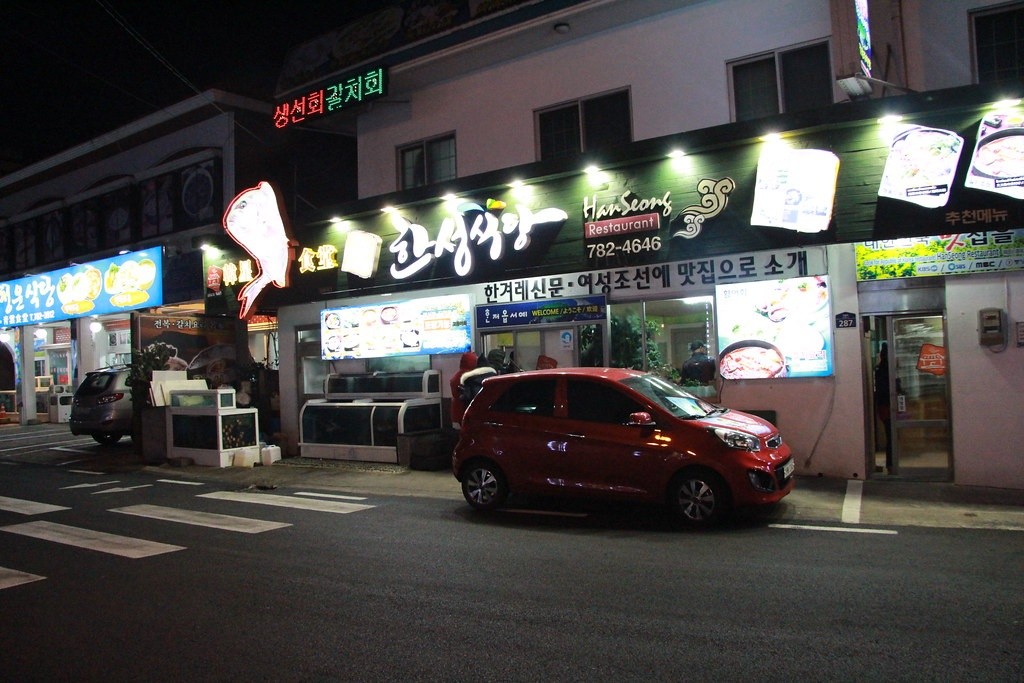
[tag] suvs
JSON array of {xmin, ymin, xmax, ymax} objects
[{"xmin": 68, "ymin": 364, "xmax": 136, "ymax": 444}]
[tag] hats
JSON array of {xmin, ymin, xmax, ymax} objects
[
  {"xmin": 880, "ymin": 343, "xmax": 888, "ymax": 358},
  {"xmin": 691, "ymin": 340, "xmax": 703, "ymax": 351}
]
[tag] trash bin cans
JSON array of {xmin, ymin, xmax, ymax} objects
[{"xmin": 50, "ymin": 385, "xmax": 74, "ymax": 423}]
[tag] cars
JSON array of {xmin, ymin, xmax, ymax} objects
[{"xmin": 452, "ymin": 367, "xmax": 797, "ymax": 531}]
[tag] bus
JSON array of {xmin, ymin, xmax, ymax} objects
[{"xmin": 0, "ymin": 388, "xmax": 50, "ymax": 422}]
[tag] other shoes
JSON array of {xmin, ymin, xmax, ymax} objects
[{"xmin": 887, "ymin": 467, "xmax": 898, "ymax": 475}]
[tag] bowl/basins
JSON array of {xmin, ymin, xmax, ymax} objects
[
  {"xmin": 719, "ymin": 340, "xmax": 789, "ymax": 378},
  {"xmin": 974, "ymin": 127, "xmax": 1024, "ymax": 178},
  {"xmin": 381, "ymin": 306, "xmax": 397, "ymax": 322}
]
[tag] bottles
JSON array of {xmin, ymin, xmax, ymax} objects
[
  {"xmin": 233, "ymin": 448, "xmax": 254, "ymax": 467},
  {"xmin": 261, "ymin": 445, "xmax": 281, "ymax": 466}
]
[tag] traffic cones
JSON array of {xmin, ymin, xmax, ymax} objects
[{"xmin": 0, "ymin": 403, "xmax": 7, "ymax": 418}]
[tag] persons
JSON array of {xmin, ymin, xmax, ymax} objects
[
  {"xmin": 873, "ymin": 341, "xmax": 903, "ymax": 474},
  {"xmin": 448, "ymin": 347, "xmax": 514, "ymax": 444},
  {"xmin": 680, "ymin": 340, "xmax": 716, "ymax": 385}
]
